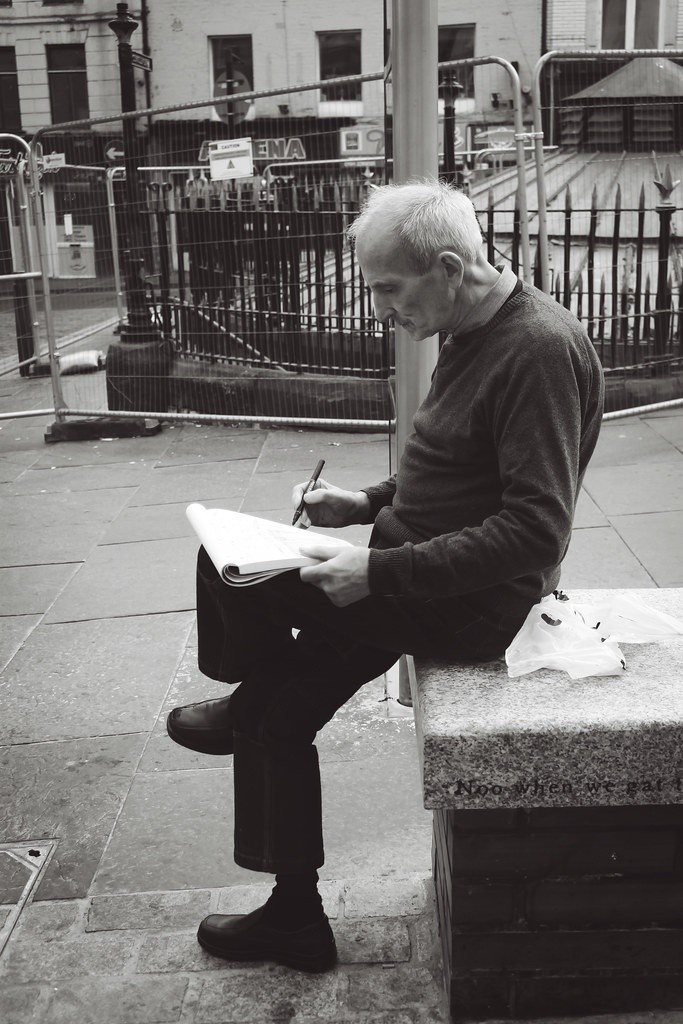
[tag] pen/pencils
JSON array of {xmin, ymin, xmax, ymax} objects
[{"xmin": 291, "ymin": 460, "xmax": 326, "ymax": 526}]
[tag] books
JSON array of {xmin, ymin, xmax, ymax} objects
[{"xmin": 185, "ymin": 504, "xmax": 357, "ymax": 587}]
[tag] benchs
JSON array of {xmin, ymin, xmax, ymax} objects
[{"xmin": 404, "ymin": 587, "xmax": 683, "ymax": 1024}]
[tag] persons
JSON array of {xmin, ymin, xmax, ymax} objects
[{"xmin": 168, "ymin": 179, "xmax": 606, "ymax": 974}]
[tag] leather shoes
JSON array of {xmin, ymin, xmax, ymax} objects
[
  {"xmin": 167, "ymin": 695, "xmax": 234, "ymax": 755},
  {"xmin": 197, "ymin": 903, "xmax": 337, "ymax": 974}
]
